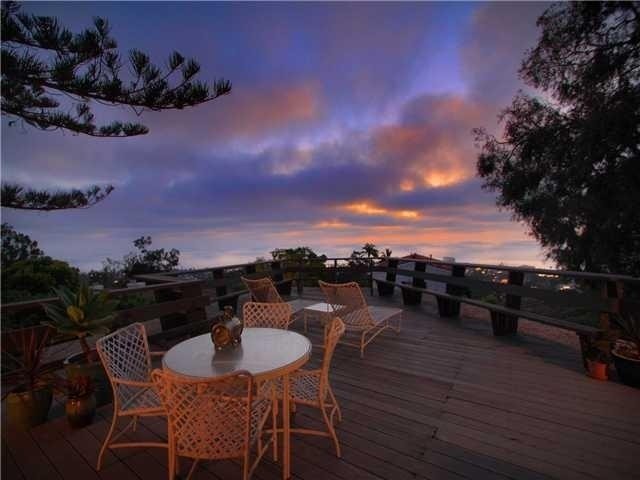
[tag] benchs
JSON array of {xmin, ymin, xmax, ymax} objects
[{"xmin": 373, "ymin": 278, "xmax": 604, "ymax": 369}]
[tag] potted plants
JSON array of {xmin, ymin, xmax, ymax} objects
[
  {"xmin": 2, "ymin": 278, "xmax": 124, "ymax": 429},
  {"xmin": 574, "ymin": 295, "xmax": 640, "ymax": 388}
]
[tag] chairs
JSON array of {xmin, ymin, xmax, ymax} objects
[
  {"xmin": 241, "ymin": 275, "xmax": 403, "ymax": 360},
  {"xmin": 96, "ymin": 322, "xmax": 175, "ymax": 480},
  {"xmin": 151, "ymin": 368, "xmax": 278, "ymax": 479},
  {"xmin": 244, "ymin": 302, "xmax": 297, "ymax": 415},
  {"xmin": 258, "ymin": 316, "xmax": 346, "ymax": 459}
]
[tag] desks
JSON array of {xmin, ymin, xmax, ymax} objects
[{"xmin": 163, "ymin": 327, "xmax": 312, "ymax": 479}]
